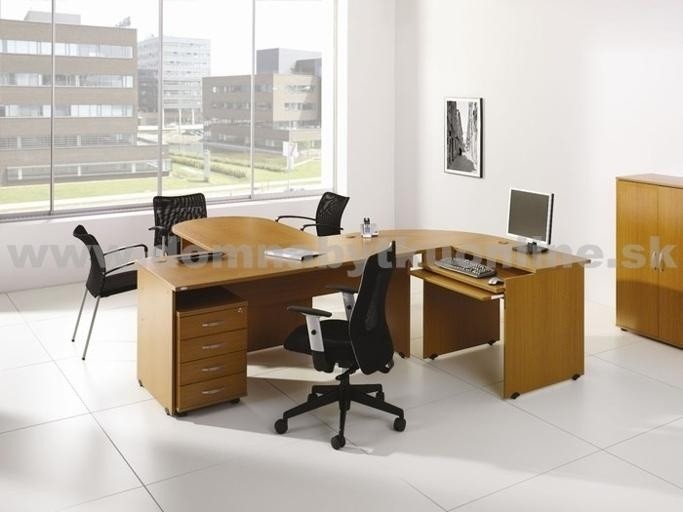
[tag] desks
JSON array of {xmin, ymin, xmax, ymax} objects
[
  {"xmin": 171, "ymin": 215, "xmax": 316, "ymax": 254},
  {"xmin": 137, "ymin": 228, "xmax": 590, "ymax": 417}
]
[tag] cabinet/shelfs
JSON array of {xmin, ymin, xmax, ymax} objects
[
  {"xmin": 615, "ymin": 172, "xmax": 682, "ymax": 351},
  {"xmin": 177, "ymin": 286, "xmax": 248, "ymax": 413}
]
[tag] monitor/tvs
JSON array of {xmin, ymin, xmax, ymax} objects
[{"xmin": 505, "ymin": 188, "xmax": 553, "ymax": 255}]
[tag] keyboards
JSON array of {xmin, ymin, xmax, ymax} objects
[{"xmin": 436, "ymin": 257, "xmax": 496, "ymax": 279}]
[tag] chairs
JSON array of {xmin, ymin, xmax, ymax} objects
[
  {"xmin": 274, "ymin": 192, "xmax": 351, "ymax": 238},
  {"xmin": 273, "ymin": 239, "xmax": 405, "ymax": 449},
  {"xmin": 149, "ymin": 193, "xmax": 207, "ymax": 255},
  {"xmin": 71, "ymin": 225, "xmax": 147, "ymax": 360}
]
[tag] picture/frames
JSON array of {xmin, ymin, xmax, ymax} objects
[{"xmin": 443, "ymin": 97, "xmax": 482, "ymax": 179}]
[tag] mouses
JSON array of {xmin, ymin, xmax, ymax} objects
[{"xmin": 488, "ymin": 275, "xmax": 503, "ymax": 285}]
